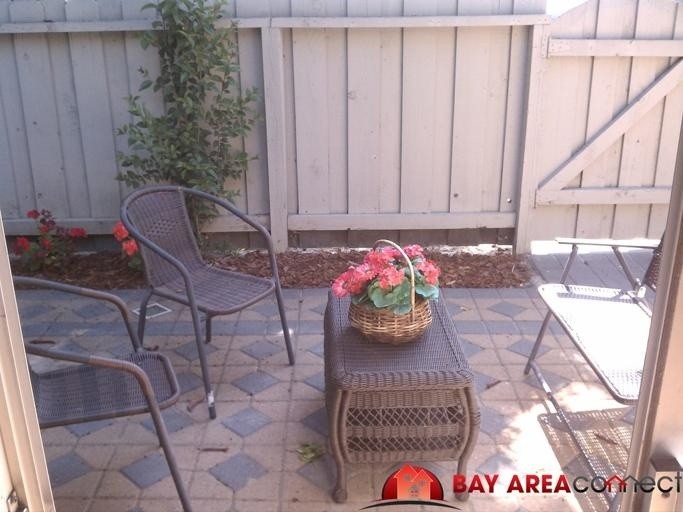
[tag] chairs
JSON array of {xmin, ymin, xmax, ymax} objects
[
  {"xmin": 523, "ymin": 225, "xmax": 668, "ymax": 512},
  {"xmin": 9, "ymin": 186, "xmax": 296, "ymax": 512}
]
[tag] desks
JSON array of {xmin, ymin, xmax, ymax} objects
[{"xmin": 323, "ymin": 289, "xmax": 481, "ymax": 502}]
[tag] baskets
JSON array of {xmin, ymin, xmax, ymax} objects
[{"xmin": 345, "ymin": 239, "xmax": 433, "ymax": 346}]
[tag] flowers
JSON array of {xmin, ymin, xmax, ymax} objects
[{"xmin": 331, "ymin": 243, "xmax": 442, "ymax": 316}]
